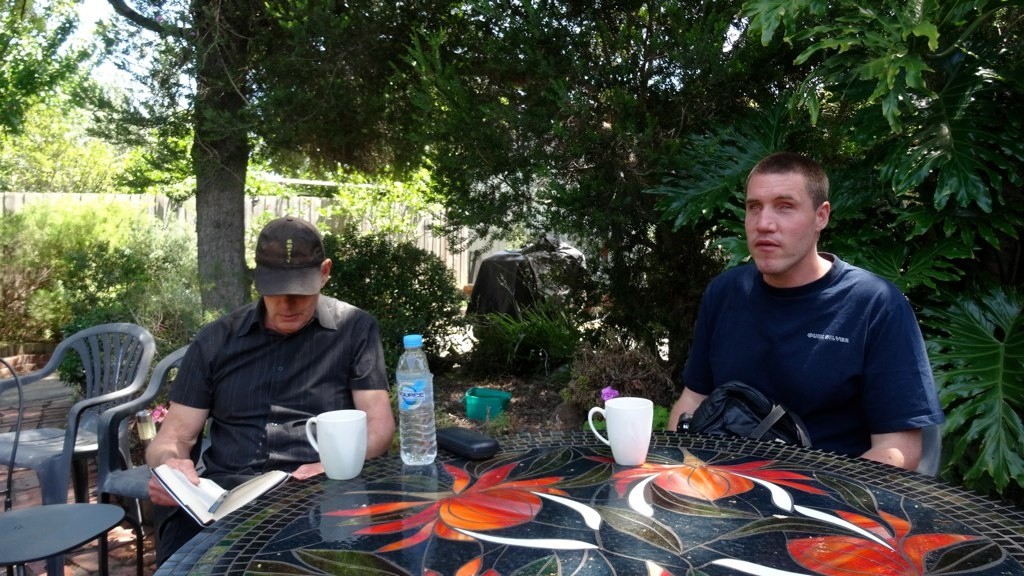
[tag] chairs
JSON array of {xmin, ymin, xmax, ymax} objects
[
  {"xmin": 97, "ymin": 344, "xmax": 213, "ymax": 576},
  {"xmin": 0, "ymin": 359, "xmax": 143, "ymax": 576},
  {"xmin": 0, "ymin": 322, "xmax": 156, "ymax": 576}
]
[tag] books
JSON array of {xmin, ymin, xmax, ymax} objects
[{"xmin": 152, "ymin": 464, "xmax": 291, "ymax": 525}]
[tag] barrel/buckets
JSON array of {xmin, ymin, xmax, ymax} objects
[{"xmin": 465, "ymin": 387, "xmax": 511, "ymax": 420}]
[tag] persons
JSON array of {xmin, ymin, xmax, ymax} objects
[
  {"xmin": 146, "ymin": 219, "xmax": 395, "ymax": 565},
  {"xmin": 667, "ymin": 152, "xmax": 943, "ymax": 470}
]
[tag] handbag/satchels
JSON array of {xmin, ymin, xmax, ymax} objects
[{"xmin": 675, "ymin": 380, "xmax": 812, "ymax": 450}]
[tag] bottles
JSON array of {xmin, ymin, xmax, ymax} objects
[
  {"xmin": 396, "ymin": 334, "xmax": 437, "ymax": 466},
  {"xmin": 137, "ymin": 409, "xmax": 157, "ymax": 445}
]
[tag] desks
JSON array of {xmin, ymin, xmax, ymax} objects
[{"xmin": 150, "ymin": 431, "xmax": 1024, "ymax": 576}]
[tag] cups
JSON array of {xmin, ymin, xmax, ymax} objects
[
  {"xmin": 588, "ymin": 397, "xmax": 654, "ymax": 466},
  {"xmin": 305, "ymin": 409, "xmax": 367, "ymax": 480}
]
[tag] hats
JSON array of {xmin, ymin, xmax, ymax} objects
[{"xmin": 255, "ymin": 217, "xmax": 326, "ymax": 295}]
